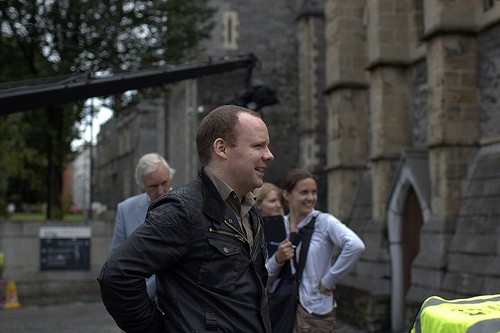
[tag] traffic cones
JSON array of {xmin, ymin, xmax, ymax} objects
[{"xmin": 3, "ymin": 280, "xmax": 23, "ymax": 310}]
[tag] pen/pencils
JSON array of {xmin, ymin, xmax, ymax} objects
[{"xmin": 269, "ymin": 239, "xmax": 298, "ymax": 249}]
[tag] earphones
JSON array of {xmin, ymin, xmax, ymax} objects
[{"xmin": 219, "ymin": 144, "xmax": 225, "ymax": 152}]
